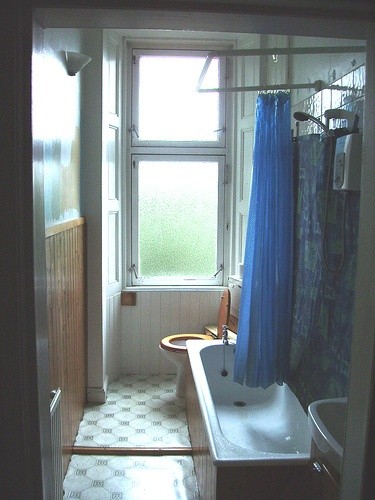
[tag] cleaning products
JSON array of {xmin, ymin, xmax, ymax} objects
[{"xmin": 238, "ymin": 264, "xmax": 244, "ymax": 279}]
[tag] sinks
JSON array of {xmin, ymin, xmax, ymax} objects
[{"xmin": 308, "ymin": 397, "xmax": 347, "ymax": 474}]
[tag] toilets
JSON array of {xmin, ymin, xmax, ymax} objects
[{"xmin": 159, "ymin": 275, "xmax": 243, "ymax": 398}]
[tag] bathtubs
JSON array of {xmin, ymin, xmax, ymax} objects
[{"xmin": 186, "ymin": 338, "xmax": 311, "ymax": 467}]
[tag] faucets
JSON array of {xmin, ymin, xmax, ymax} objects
[{"xmin": 222, "ymin": 325, "xmax": 228, "ymax": 347}]
